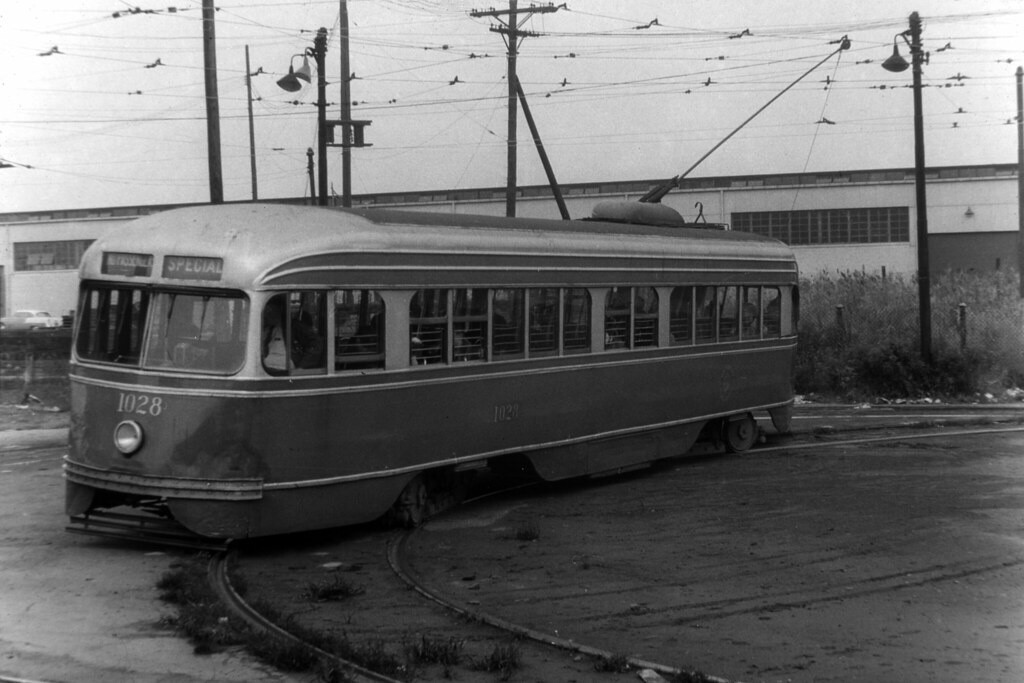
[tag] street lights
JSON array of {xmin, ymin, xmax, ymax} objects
[
  {"xmin": 881, "ymin": 12, "xmax": 933, "ymax": 366},
  {"xmin": 276, "ymin": 28, "xmax": 328, "ymax": 205}
]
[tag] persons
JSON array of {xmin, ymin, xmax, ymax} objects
[
  {"xmin": 262, "ymin": 297, "xmax": 301, "ymax": 370},
  {"xmin": 729, "ymin": 301, "xmax": 769, "ymax": 335}
]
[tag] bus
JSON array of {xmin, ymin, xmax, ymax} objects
[{"xmin": 63, "ymin": 202, "xmax": 801, "ymax": 551}]
